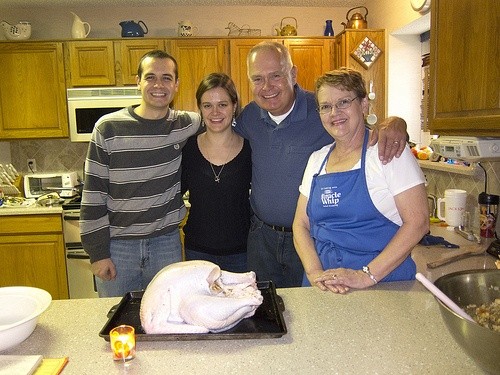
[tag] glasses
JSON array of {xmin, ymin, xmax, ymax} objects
[{"xmin": 315, "ymin": 96, "xmax": 357, "ymax": 113}]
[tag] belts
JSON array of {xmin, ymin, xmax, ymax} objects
[{"xmin": 253, "ymin": 212, "xmax": 292, "ymax": 232}]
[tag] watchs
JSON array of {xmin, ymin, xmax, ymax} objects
[{"xmin": 362, "ymin": 266, "xmax": 378, "ymax": 285}]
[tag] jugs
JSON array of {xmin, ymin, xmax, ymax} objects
[
  {"xmin": 436, "ymin": 188, "xmax": 471, "ymax": 228},
  {"xmin": 118, "ymin": 19, "xmax": 149, "ymax": 37},
  {"xmin": 176, "ymin": 19, "xmax": 199, "ymax": 36}
]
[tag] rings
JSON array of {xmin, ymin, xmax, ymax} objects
[
  {"xmin": 332, "ymin": 275, "xmax": 337, "ymax": 279},
  {"xmin": 393, "ymin": 141, "xmax": 399, "ymax": 144}
]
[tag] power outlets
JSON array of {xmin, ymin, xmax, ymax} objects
[{"xmin": 27, "ymin": 158, "xmax": 36, "ymax": 172}]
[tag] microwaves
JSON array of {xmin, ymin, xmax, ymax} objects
[{"xmin": 23, "ymin": 169, "xmax": 78, "ymax": 198}]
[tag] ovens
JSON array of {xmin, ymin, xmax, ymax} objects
[
  {"xmin": 67, "ymin": 86, "xmax": 160, "ymax": 144},
  {"xmin": 63, "ymin": 242, "xmax": 104, "ymax": 298}
]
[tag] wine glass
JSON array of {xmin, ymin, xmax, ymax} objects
[{"xmin": 478, "ymin": 192, "xmax": 499, "ymax": 242}]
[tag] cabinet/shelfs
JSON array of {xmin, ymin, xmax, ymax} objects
[
  {"xmin": 0, "ymin": 213, "xmax": 69, "ymax": 299},
  {"xmin": 0, "ymin": 35, "xmax": 386, "ymax": 139},
  {"xmin": 426, "ymin": 0, "xmax": 500, "ymax": 129}
]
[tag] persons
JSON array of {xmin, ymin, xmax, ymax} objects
[
  {"xmin": 292, "ymin": 68, "xmax": 430, "ymax": 293},
  {"xmin": 79, "ymin": 51, "xmax": 240, "ymax": 299},
  {"xmin": 199, "ymin": 40, "xmax": 409, "ymax": 288},
  {"xmin": 182, "ymin": 72, "xmax": 250, "ymax": 273}
]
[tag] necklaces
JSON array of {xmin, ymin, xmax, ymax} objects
[{"xmin": 205, "ymin": 133, "xmax": 234, "ymax": 182}]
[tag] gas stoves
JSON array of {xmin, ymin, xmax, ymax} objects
[{"xmin": 61, "ymin": 157, "xmax": 110, "ymax": 255}]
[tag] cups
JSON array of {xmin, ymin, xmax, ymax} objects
[
  {"xmin": 71, "ymin": 12, "xmax": 91, "ymax": 39},
  {"xmin": 110, "ymin": 325, "xmax": 136, "ymax": 364}
]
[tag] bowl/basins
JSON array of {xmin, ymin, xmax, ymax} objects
[
  {"xmin": 1, "ymin": 286, "xmax": 53, "ymax": 350},
  {"xmin": 432, "ymin": 268, "xmax": 500, "ymax": 375},
  {"xmin": 0, "ymin": 20, "xmax": 32, "ymax": 41}
]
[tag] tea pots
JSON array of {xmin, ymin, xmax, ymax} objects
[
  {"xmin": 274, "ymin": 16, "xmax": 298, "ymax": 36},
  {"xmin": 341, "ymin": 6, "xmax": 369, "ymax": 29}
]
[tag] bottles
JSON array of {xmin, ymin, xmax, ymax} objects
[{"xmin": 324, "ymin": 20, "xmax": 335, "ymax": 34}]
